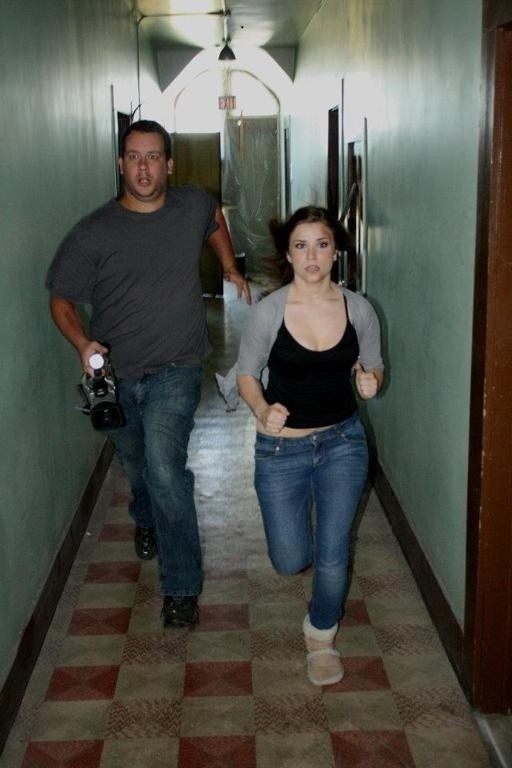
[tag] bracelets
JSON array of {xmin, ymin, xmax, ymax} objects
[{"xmin": 223, "ymin": 267, "xmax": 237, "ymax": 280}]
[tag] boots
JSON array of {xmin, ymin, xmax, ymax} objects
[{"xmin": 302, "ymin": 614, "xmax": 344, "ymax": 686}]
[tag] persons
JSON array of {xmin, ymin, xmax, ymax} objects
[
  {"xmin": 46, "ymin": 121, "xmax": 251, "ymax": 628},
  {"xmin": 215, "ymin": 206, "xmax": 385, "ymax": 687}
]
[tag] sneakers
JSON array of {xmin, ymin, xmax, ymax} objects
[
  {"xmin": 163, "ymin": 595, "xmax": 199, "ymax": 629},
  {"xmin": 136, "ymin": 526, "xmax": 156, "ymax": 562}
]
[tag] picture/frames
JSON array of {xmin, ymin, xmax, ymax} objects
[{"xmin": 344, "ymin": 117, "xmax": 366, "ymax": 297}]
